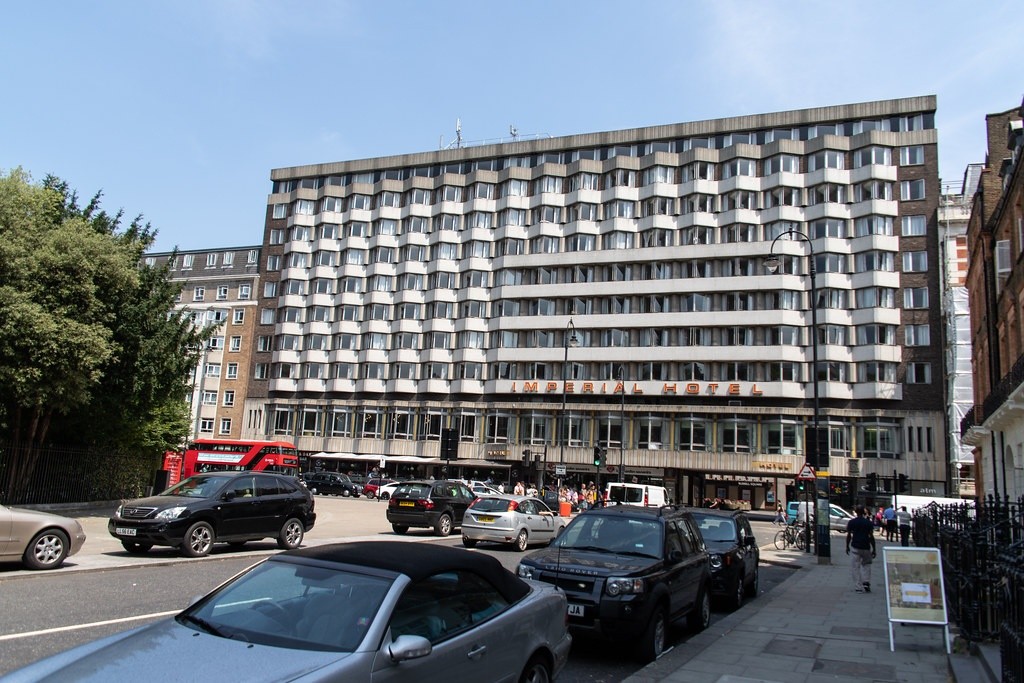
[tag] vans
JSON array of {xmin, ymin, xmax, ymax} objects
[
  {"xmin": 797, "ymin": 501, "xmax": 856, "ymax": 531},
  {"xmin": 785, "ymin": 502, "xmax": 799, "ymax": 526},
  {"xmin": 604, "ymin": 482, "xmax": 673, "ymax": 528},
  {"xmin": 447, "ymin": 479, "xmax": 508, "ymax": 505}
]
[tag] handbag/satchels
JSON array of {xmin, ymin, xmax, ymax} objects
[
  {"xmin": 880, "ymin": 519, "xmax": 887, "ymax": 526},
  {"xmin": 875, "ymin": 512, "xmax": 882, "ymax": 520},
  {"xmin": 782, "ymin": 507, "xmax": 784, "ymax": 512},
  {"xmin": 578, "ymin": 495, "xmax": 585, "ymax": 500}
]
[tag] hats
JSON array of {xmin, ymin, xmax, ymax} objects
[
  {"xmin": 530, "ymin": 485, "xmax": 536, "ymax": 488},
  {"xmin": 520, "ymin": 481, "xmax": 524, "ymax": 483},
  {"xmin": 589, "ymin": 481, "xmax": 594, "ymax": 486}
]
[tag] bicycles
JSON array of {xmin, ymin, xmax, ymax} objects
[{"xmin": 774, "ymin": 514, "xmax": 814, "ymax": 550}]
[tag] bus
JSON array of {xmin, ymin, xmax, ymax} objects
[{"xmin": 184, "ymin": 438, "xmax": 301, "ymax": 489}]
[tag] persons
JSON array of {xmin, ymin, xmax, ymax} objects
[
  {"xmin": 669, "ymin": 499, "xmax": 686, "ymax": 510},
  {"xmin": 894, "ymin": 506, "xmax": 911, "ymax": 546},
  {"xmin": 853, "ymin": 504, "xmax": 896, "ymax": 542},
  {"xmin": 846, "ymin": 505, "xmax": 876, "ymax": 593},
  {"xmin": 772, "ymin": 500, "xmax": 787, "ymax": 524},
  {"xmin": 445, "ymin": 475, "xmax": 504, "ymax": 493},
  {"xmin": 703, "ymin": 497, "xmax": 726, "ymax": 510},
  {"xmin": 514, "ymin": 481, "xmax": 602, "ymax": 513},
  {"xmin": 347, "ymin": 470, "xmax": 435, "ymax": 489},
  {"xmin": 200, "ymin": 467, "xmax": 217, "ymax": 473}
]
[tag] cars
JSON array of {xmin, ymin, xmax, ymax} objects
[
  {"xmin": 1, "ymin": 540, "xmax": 574, "ymax": 683},
  {"xmin": 544, "ymin": 491, "xmax": 577, "ymax": 512},
  {"xmin": 461, "ymin": 494, "xmax": 568, "ymax": 552},
  {"xmin": 0, "ymin": 502, "xmax": 86, "ymax": 571},
  {"xmin": 302, "ymin": 472, "xmax": 421, "ymax": 500}
]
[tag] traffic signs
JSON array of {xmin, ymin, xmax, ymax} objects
[{"xmin": 555, "ymin": 465, "xmax": 566, "ymax": 475}]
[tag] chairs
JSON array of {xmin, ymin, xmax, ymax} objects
[
  {"xmin": 597, "ymin": 521, "xmax": 620, "ymax": 548},
  {"xmin": 294, "ymin": 593, "xmax": 350, "ymax": 644},
  {"xmin": 391, "ymin": 586, "xmax": 440, "ymax": 641}
]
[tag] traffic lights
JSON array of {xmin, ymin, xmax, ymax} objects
[
  {"xmin": 593, "ymin": 446, "xmax": 603, "ymax": 466},
  {"xmin": 865, "ymin": 472, "xmax": 876, "ymax": 491},
  {"xmin": 522, "ymin": 449, "xmax": 530, "ymax": 467},
  {"xmin": 799, "ymin": 479, "xmax": 805, "ymax": 493},
  {"xmin": 899, "ymin": 473, "xmax": 909, "ymax": 493}
]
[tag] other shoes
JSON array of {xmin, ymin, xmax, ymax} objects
[
  {"xmin": 772, "ymin": 521, "xmax": 775, "ymax": 525},
  {"xmin": 863, "ymin": 582, "xmax": 870, "ymax": 592},
  {"xmin": 856, "ymin": 590, "xmax": 863, "ymax": 593}
]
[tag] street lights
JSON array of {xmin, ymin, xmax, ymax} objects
[
  {"xmin": 761, "ymin": 230, "xmax": 821, "ymax": 472},
  {"xmin": 559, "ymin": 320, "xmax": 579, "ymax": 488}
]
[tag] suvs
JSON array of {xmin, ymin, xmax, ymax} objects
[
  {"xmin": 386, "ymin": 479, "xmax": 479, "ymax": 537},
  {"xmin": 515, "ymin": 500, "xmax": 712, "ymax": 660},
  {"xmin": 108, "ymin": 470, "xmax": 316, "ymax": 558},
  {"xmin": 683, "ymin": 507, "xmax": 759, "ymax": 614}
]
